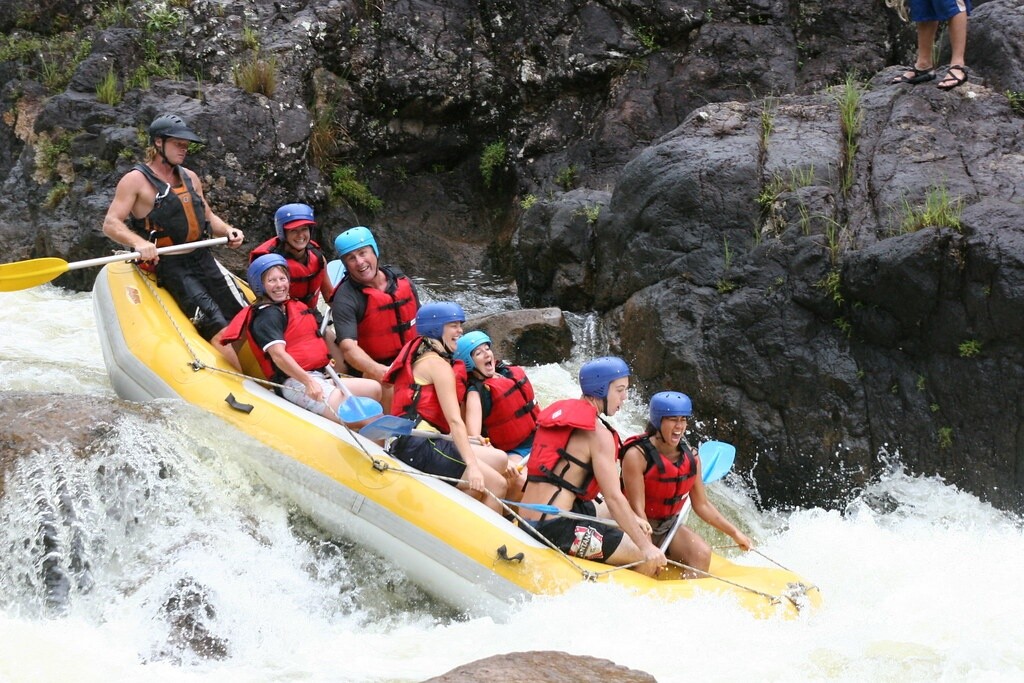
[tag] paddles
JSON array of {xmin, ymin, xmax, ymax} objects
[
  {"xmin": 358, "ymin": 415, "xmax": 482, "ymax": 445},
  {"xmin": 325, "ymin": 364, "xmax": 384, "ymax": 424},
  {"xmin": 0, "ymin": 231, "xmax": 239, "ymax": 293},
  {"xmin": 499, "ymin": 498, "xmax": 618, "ymax": 529},
  {"xmin": 658, "ymin": 441, "xmax": 737, "ymax": 554},
  {"xmin": 513, "ymin": 451, "xmax": 532, "ymax": 475},
  {"xmin": 315, "ymin": 259, "xmax": 347, "ymax": 338}
]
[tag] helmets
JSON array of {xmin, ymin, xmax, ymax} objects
[
  {"xmin": 648, "ymin": 391, "xmax": 692, "ymax": 431},
  {"xmin": 246, "ymin": 253, "xmax": 289, "ymax": 298},
  {"xmin": 273, "ymin": 202, "xmax": 316, "ymax": 242},
  {"xmin": 149, "ymin": 113, "xmax": 202, "ymax": 144},
  {"xmin": 415, "ymin": 301, "xmax": 466, "ymax": 341},
  {"xmin": 580, "ymin": 356, "xmax": 630, "ymax": 398},
  {"xmin": 453, "ymin": 330, "xmax": 491, "ymax": 372},
  {"xmin": 334, "ymin": 226, "xmax": 379, "ymax": 268}
]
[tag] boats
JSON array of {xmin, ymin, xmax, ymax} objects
[{"xmin": 90, "ymin": 260, "xmax": 822, "ymax": 623}]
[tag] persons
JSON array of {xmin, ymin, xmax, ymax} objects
[
  {"xmin": 892, "ymin": 0, "xmax": 969, "ymax": 90},
  {"xmin": 453, "ymin": 331, "xmax": 540, "ymax": 523},
  {"xmin": 248, "ymin": 254, "xmax": 381, "ymax": 429},
  {"xmin": 519, "ymin": 357, "xmax": 668, "ymax": 578},
  {"xmin": 102, "ymin": 113, "xmax": 243, "ymax": 339},
  {"xmin": 616, "ymin": 392, "xmax": 750, "ymax": 579},
  {"xmin": 380, "ymin": 302, "xmax": 507, "ymax": 517},
  {"xmin": 249, "ymin": 203, "xmax": 332, "ymax": 329},
  {"xmin": 329, "ymin": 227, "xmax": 422, "ymax": 415}
]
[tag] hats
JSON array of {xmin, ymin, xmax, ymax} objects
[{"xmin": 283, "ymin": 219, "xmax": 316, "ymax": 230}]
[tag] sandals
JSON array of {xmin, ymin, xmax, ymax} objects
[
  {"xmin": 891, "ymin": 65, "xmax": 936, "ymax": 85},
  {"xmin": 937, "ymin": 65, "xmax": 968, "ymax": 89}
]
